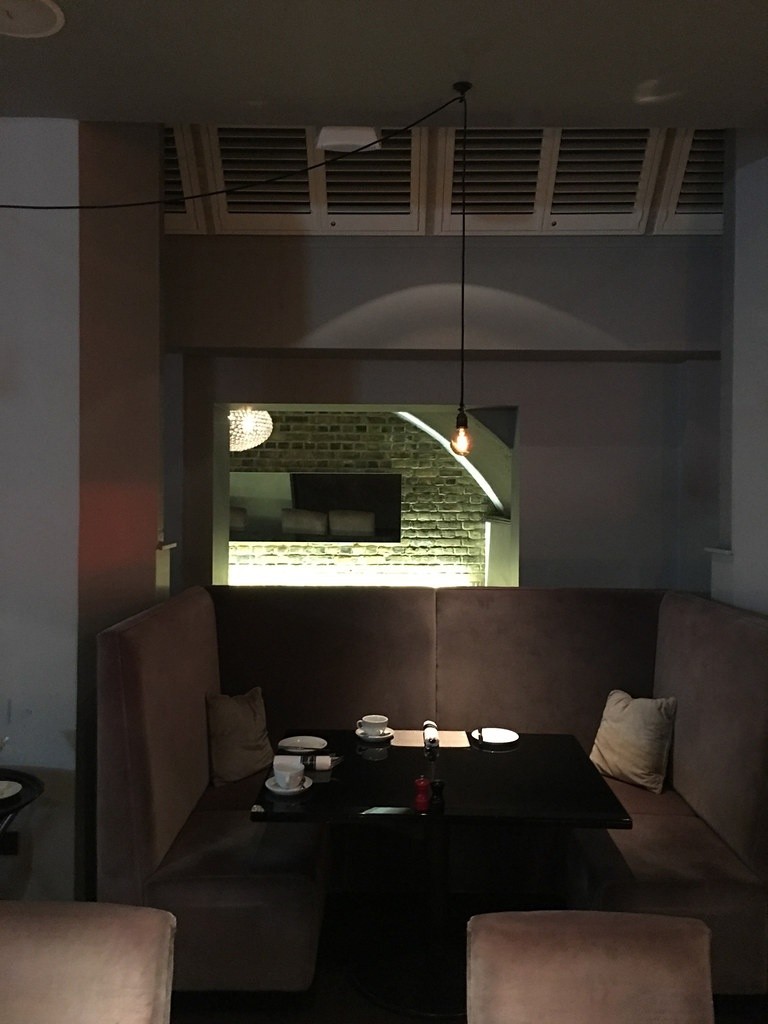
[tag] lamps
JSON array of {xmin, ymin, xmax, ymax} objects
[{"xmin": 450, "ymin": 80, "xmax": 474, "ymax": 455}]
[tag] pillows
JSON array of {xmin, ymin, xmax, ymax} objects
[
  {"xmin": 588, "ymin": 690, "xmax": 678, "ymax": 795},
  {"xmin": 207, "ymin": 685, "xmax": 275, "ymax": 787}
]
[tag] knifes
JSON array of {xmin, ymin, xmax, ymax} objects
[{"xmin": 278, "ymin": 745, "xmax": 328, "ymax": 753}]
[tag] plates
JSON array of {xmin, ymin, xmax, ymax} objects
[
  {"xmin": 265, "ymin": 775, "xmax": 313, "ymax": 795},
  {"xmin": 356, "ymin": 726, "xmax": 394, "ymax": 741},
  {"xmin": 471, "ymin": 727, "xmax": 520, "ymax": 744},
  {"xmin": 278, "ymin": 736, "xmax": 328, "ymax": 753}
]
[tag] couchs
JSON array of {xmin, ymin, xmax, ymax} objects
[
  {"xmin": 436, "ymin": 586, "xmax": 768, "ymax": 994},
  {"xmin": 96, "ymin": 586, "xmax": 436, "ymax": 992}
]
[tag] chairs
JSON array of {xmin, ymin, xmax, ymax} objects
[
  {"xmin": 467, "ymin": 910, "xmax": 713, "ymax": 1024},
  {"xmin": 0, "ymin": 901, "xmax": 177, "ymax": 1024}
]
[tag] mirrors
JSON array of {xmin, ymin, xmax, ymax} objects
[{"xmin": 212, "ymin": 403, "xmax": 522, "ymax": 588}]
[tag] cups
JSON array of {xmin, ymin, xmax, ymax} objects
[
  {"xmin": 356, "ymin": 715, "xmax": 388, "ymax": 735},
  {"xmin": 273, "ymin": 760, "xmax": 305, "ymax": 788}
]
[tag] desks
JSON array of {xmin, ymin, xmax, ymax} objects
[{"xmin": 249, "ymin": 729, "xmax": 635, "ymax": 899}]
